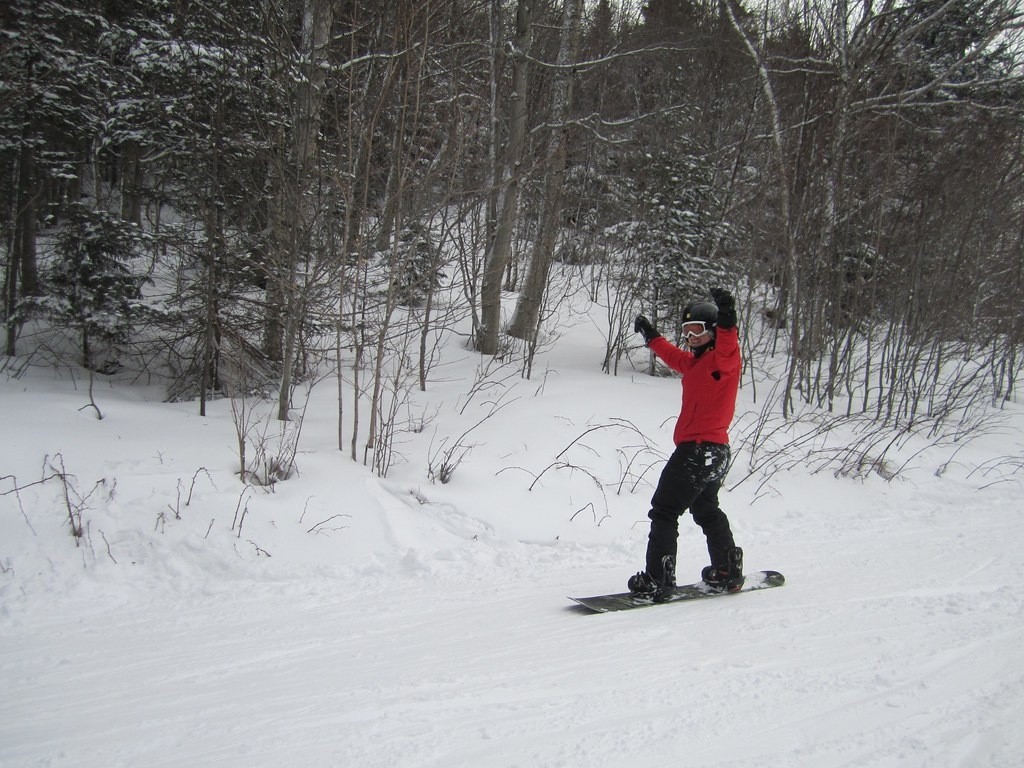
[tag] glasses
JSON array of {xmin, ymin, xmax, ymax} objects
[{"xmin": 683, "ymin": 321, "xmax": 708, "ymax": 338}]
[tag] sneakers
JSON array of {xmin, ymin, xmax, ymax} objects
[
  {"xmin": 628, "ymin": 556, "xmax": 677, "ymax": 598},
  {"xmin": 702, "ymin": 546, "xmax": 744, "ymax": 591}
]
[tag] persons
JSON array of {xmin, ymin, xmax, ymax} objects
[{"xmin": 628, "ymin": 287, "xmax": 741, "ymax": 592}]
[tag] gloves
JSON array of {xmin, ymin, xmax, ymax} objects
[
  {"xmin": 634, "ymin": 316, "xmax": 661, "ymax": 348},
  {"xmin": 710, "ymin": 288, "xmax": 737, "ymax": 329}
]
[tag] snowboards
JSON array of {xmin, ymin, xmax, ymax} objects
[{"xmin": 565, "ymin": 570, "xmax": 786, "ymax": 614}]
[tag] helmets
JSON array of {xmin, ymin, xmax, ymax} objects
[{"xmin": 682, "ymin": 301, "xmax": 719, "ymax": 332}]
